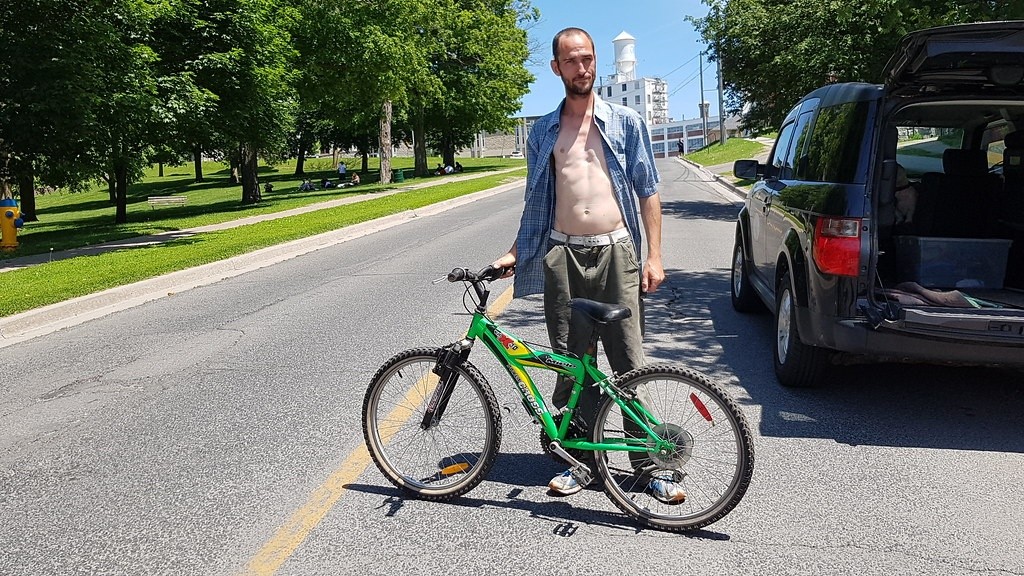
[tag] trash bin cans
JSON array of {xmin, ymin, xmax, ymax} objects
[
  {"xmin": 391, "ymin": 167, "xmax": 405, "ymax": 183},
  {"xmin": 0, "ymin": 198, "xmax": 26, "ymax": 253}
]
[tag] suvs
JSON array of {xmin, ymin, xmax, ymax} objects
[{"xmin": 731, "ymin": 16, "xmax": 1024, "ymax": 389}]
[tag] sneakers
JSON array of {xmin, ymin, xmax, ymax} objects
[
  {"xmin": 634, "ymin": 470, "xmax": 687, "ymax": 503},
  {"xmin": 548, "ymin": 461, "xmax": 600, "ymax": 494}
]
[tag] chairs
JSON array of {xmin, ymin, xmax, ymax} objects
[
  {"xmin": 1003, "ymin": 127, "xmax": 1024, "ymax": 222},
  {"xmin": 912, "ymin": 147, "xmax": 1000, "ymax": 235}
]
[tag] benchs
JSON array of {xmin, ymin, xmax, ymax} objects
[{"xmin": 148, "ymin": 195, "xmax": 187, "ymax": 210}]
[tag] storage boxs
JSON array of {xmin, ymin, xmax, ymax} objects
[{"xmin": 891, "ymin": 235, "xmax": 1014, "ymax": 289}]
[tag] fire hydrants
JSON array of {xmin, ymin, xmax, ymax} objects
[{"xmin": 0, "ymin": 193, "xmax": 26, "ymax": 248}]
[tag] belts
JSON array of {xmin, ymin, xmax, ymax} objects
[{"xmin": 550, "ymin": 227, "xmax": 629, "ymax": 247}]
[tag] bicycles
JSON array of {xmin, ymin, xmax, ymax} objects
[{"xmin": 361, "ymin": 264, "xmax": 756, "ymax": 533}]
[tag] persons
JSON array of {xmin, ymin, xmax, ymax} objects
[
  {"xmin": 433, "ymin": 161, "xmax": 463, "ymax": 176},
  {"xmin": 677, "ymin": 139, "xmax": 684, "ymax": 157},
  {"xmin": 336, "ymin": 161, "xmax": 346, "ymax": 180},
  {"xmin": 264, "ymin": 180, "xmax": 274, "ymax": 192},
  {"xmin": 350, "ymin": 171, "xmax": 360, "ymax": 184},
  {"xmin": 298, "ymin": 178, "xmax": 315, "ymax": 192},
  {"xmin": 320, "ymin": 176, "xmax": 335, "ymax": 189},
  {"xmin": 491, "ymin": 27, "xmax": 687, "ymax": 503}
]
